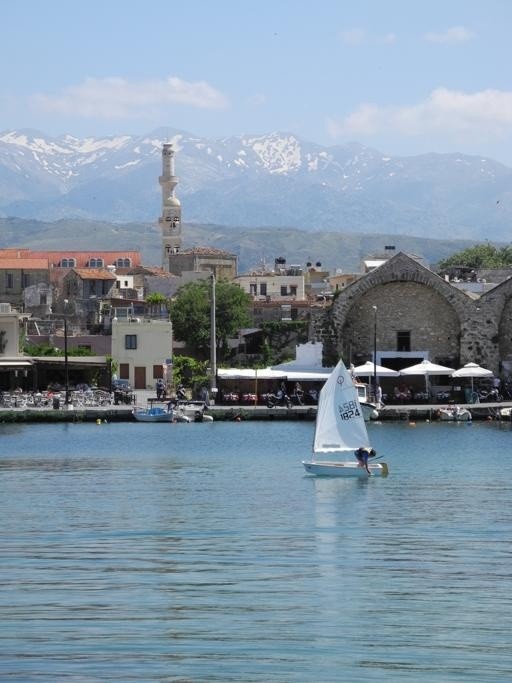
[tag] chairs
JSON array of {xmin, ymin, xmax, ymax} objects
[{"xmin": 0, "ymin": 387, "xmax": 136, "ymax": 407}]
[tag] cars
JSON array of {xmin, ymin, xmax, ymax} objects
[{"xmin": 113, "ymin": 380, "xmax": 131, "ymax": 390}]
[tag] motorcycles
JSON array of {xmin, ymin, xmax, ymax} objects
[
  {"xmin": 265, "ymin": 392, "xmax": 292, "ymax": 408},
  {"xmin": 476, "ymin": 383, "xmax": 512, "ymax": 402},
  {"xmin": 156, "ymin": 383, "xmax": 186, "ymax": 402}
]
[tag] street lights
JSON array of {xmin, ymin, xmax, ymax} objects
[
  {"xmin": 57, "ymin": 317, "xmax": 68, "ymax": 407},
  {"xmin": 372, "ymin": 304, "xmax": 377, "ymax": 404}
]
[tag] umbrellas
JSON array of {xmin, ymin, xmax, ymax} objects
[
  {"xmin": 447, "ymin": 360, "xmax": 494, "ymax": 393},
  {"xmin": 344, "ymin": 360, "xmax": 401, "ymax": 396},
  {"xmin": 398, "ymin": 358, "xmax": 454, "ymax": 390}
]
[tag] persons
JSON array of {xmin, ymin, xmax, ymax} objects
[
  {"xmin": 202, "ymin": 386, "xmax": 212, "ymax": 405},
  {"xmin": 176, "ymin": 382, "xmax": 186, "ymax": 404},
  {"xmin": 355, "ymin": 446, "xmax": 377, "ymax": 475},
  {"xmin": 155, "ymin": 378, "xmax": 165, "ymax": 401},
  {"xmin": 9, "ymin": 378, "xmax": 133, "ymax": 410},
  {"xmin": 216, "ymin": 376, "xmax": 511, "ymax": 408}
]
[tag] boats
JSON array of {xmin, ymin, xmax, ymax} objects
[
  {"xmin": 131, "ymin": 398, "xmax": 213, "ymax": 423},
  {"xmin": 301, "ymin": 357, "xmax": 388, "ymax": 477},
  {"xmin": 437, "ymin": 407, "xmax": 512, "ymax": 421},
  {"xmin": 347, "ymin": 362, "xmax": 376, "ymax": 424}
]
